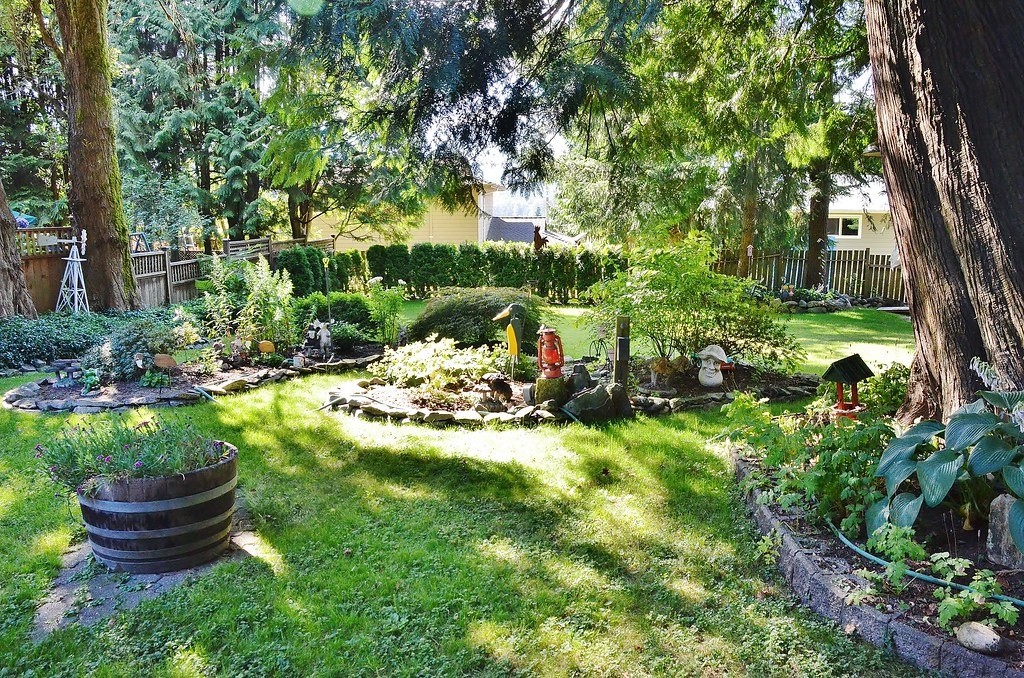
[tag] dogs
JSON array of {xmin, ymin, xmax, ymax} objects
[{"xmin": 490, "ymin": 378, "xmax": 512, "ymax": 402}]
